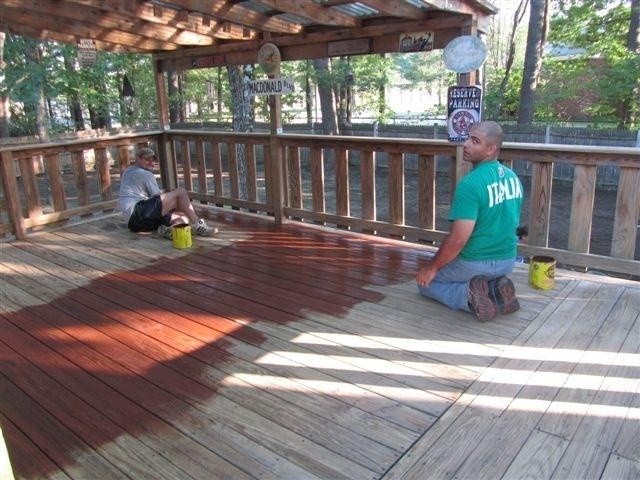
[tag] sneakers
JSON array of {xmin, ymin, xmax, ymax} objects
[
  {"xmin": 495, "ymin": 277, "xmax": 519, "ymax": 315},
  {"xmin": 468, "ymin": 275, "xmax": 496, "ymax": 322},
  {"xmin": 191, "ymin": 219, "xmax": 218, "ymax": 236},
  {"xmin": 158, "ymin": 225, "xmax": 173, "ymax": 240}
]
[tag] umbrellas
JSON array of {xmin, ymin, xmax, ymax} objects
[{"xmin": 121, "ymin": 74, "xmax": 135, "ymax": 96}]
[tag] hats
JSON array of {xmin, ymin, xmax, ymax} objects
[{"xmin": 137, "ymin": 148, "xmax": 157, "ymax": 160}]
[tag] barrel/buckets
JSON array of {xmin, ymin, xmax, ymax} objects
[
  {"xmin": 529, "ymin": 256, "xmax": 556, "ymax": 290},
  {"xmin": 172, "ymin": 225, "xmax": 193, "ymax": 249}
]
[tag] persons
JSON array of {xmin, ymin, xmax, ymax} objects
[
  {"xmin": 117, "ymin": 148, "xmax": 219, "ymax": 242},
  {"xmin": 94, "ymin": 111, "xmax": 106, "ymax": 128},
  {"xmin": 416, "ymin": 119, "xmax": 524, "ymax": 323}
]
